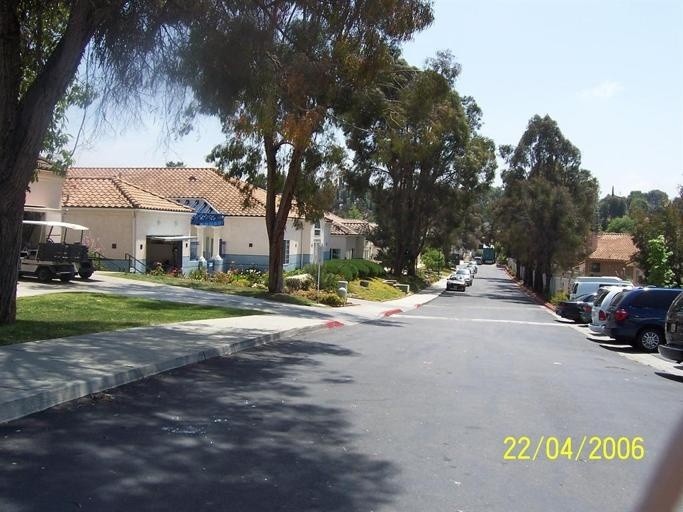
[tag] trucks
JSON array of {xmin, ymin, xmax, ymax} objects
[{"xmin": 482, "ymin": 248, "xmax": 495, "ymax": 264}]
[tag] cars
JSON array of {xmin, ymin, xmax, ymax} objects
[{"xmin": 447, "ymin": 260, "xmax": 478, "ymax": 292}]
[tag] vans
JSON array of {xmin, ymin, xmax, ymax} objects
[{"xmin": 473, "ymin": 256, "xmax": 482, "ymax": 265}]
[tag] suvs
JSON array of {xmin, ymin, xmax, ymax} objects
[{"xmin": 555, "ymin": 276, "xmax": 683, "ymax": 362}]
